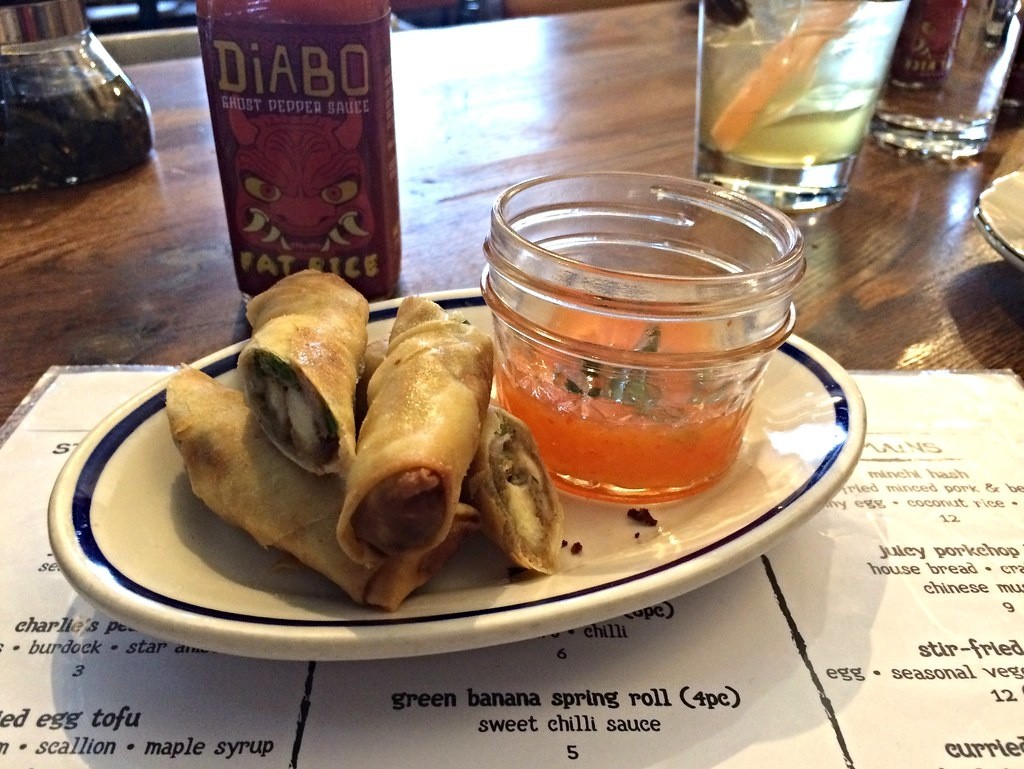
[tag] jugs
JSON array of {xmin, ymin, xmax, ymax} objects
[{"xmin": 0, "ymin": 0, "xmax": 155, "ymax": 192}]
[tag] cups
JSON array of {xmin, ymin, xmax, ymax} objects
[
  {"xmin": 694, "ymin": 1, "xmax": 912, "ymax": 211},
  {"xmin": 869, "ymin": 0, "xmax": 1024, "ymax": 160},
  {"xmin": 478, "ymin": 168, "xmax": 809, "ymax": 507}
]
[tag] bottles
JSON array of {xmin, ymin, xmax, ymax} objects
[
  {"xmin": 890, "ymin": 0, "xmax": 971, "ymax": 89},
  {"xmin": 195, "ymin": 1, "xmax": 402, "ymax": 307}
]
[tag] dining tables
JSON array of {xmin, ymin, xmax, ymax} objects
[{"xmin": 0, "ymin": 0, "xmax": 1024, "ymax": 769}]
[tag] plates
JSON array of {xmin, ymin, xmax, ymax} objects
[
  {"xmin": 973, "ymin": 162, "xmax": 1024, "ymax": 269},
  {"xmin": 47, "ymin": 286, "xmax": 868, "ymax": 663}
]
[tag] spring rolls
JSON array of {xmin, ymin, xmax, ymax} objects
[{"xmin": 166, "ymin": 266, "xmax": 565, "ymax": 608}]
